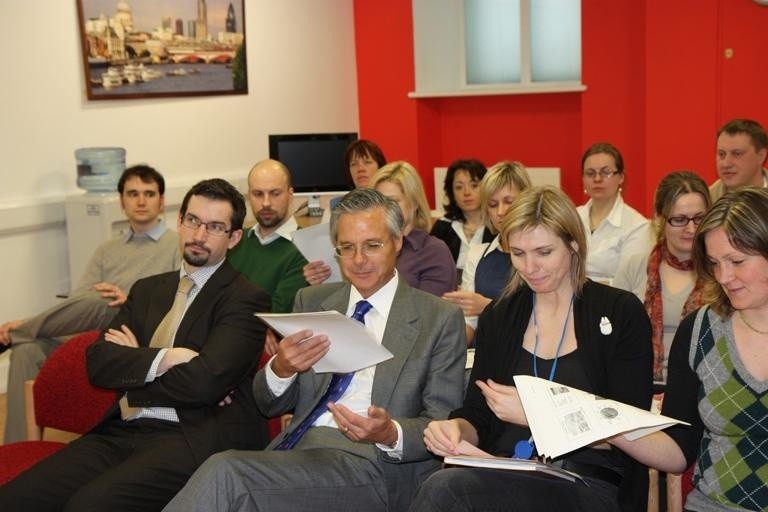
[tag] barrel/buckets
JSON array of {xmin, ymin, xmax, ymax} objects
[{"xmin": 74, "ymin": 147, "xmax": 126, "ymax": 193}]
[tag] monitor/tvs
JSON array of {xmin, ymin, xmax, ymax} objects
[{"xmin": 269, "ymin": 132, "xmax": 358, "ymax": 207}]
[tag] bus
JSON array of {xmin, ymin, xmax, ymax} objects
[{"xmin": 213, "ymin": 43, "xmax": 232, "ymax": 51}]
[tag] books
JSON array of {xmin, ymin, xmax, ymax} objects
[
  {"xmin": 443, "ymin": 454, "xmax": 592, "ymax": 491},
  {"xmin": 512, "ymin": 371, "xmax": 693, "ymax": 459}
]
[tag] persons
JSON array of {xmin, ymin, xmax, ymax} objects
[
  {"xmin": 156, "ymin": 187, "xmax": 468, "ymax": 512},
  {"xmin": 0, "ymin": 117, "xmax": 768, "ymax": 352},
  {"xmin": 0, "ymin": 176, "xmax": 272, "ymax": 512},
  {"xmin": 599, "ymin": 186, "xmax": 767, "ymax": 511},
  {"xmin": 406, "ymin": 183, "xmax": 656, "ymax": 512}
]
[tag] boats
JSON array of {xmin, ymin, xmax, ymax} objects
[
  {"xmin": 88, "ymin": 55, "xmax": 111, "ymax": 69},
  {"xmin": 166, "ymin": 68, "xmax": 187, "ymax": 77},
  {"xmin": 188, "ymin": 67, "xmax": 200, "ymax": 75},
  {"xmin": 225, "ymin": 63, "xmax": 233, "ymax": 69}
]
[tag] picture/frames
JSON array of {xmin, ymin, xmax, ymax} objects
[{"xmin": 77, "ymin": 1, "xmax": 248, "ymax": 101}]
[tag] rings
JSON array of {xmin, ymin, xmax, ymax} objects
[{"xmin": 341, "ymin": 428, "xmax": 349, "ymax": 434}]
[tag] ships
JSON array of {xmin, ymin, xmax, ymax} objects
[{"xmin": 90, "ymin": 63, "xmax": 162, "ymax": 89}]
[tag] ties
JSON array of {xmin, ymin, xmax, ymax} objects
[
  {"xmin": 118, "ymin": 276, "xmax": 194, "ymax": 421},
  {"xmin": 273, "ymin": 300, "xmax": 373, "ymax": 450}
]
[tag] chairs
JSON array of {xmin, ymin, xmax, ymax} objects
[
  {"xmin": 255, "ymin": 351, "xmax": 285, "ymax": 445},
  {"xmin": 0, "ymin": 333, "xmax": 117, "ymax": 512}
]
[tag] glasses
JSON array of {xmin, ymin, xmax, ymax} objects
[
  {"xmin": 181, "ymin": 209, "xmax": 234, "ymax": 238},
  {"xmin": 663, "ymin": 213, "xmax": 704, "ymax": 228},
  {"xmin": 583, "ymin": 167, "xmax": 620, "ymax": 180},
  {"xmin": 332, "ymin": 235, "xmax": 392, "ymax": 260}
]
